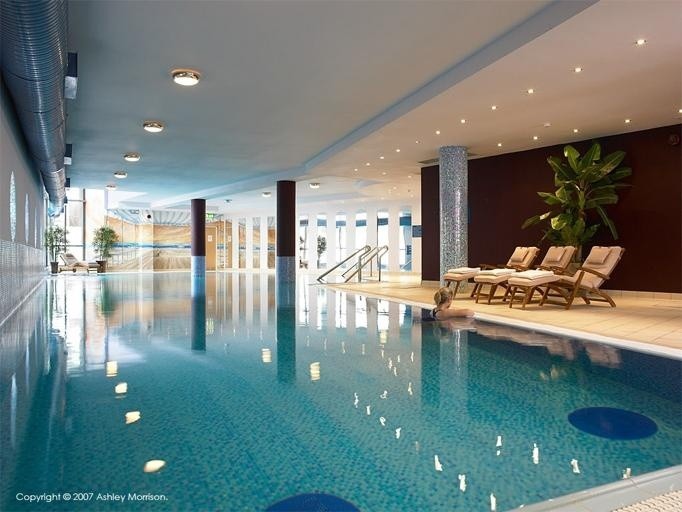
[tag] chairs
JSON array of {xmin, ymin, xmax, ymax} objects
[
  {"xmin": 442, "ymin": 246, "xmax": 626, "ymax": 309},
  {"xmin": 59, "ymin": 254, "xmax": 100, "ymax": 274}
]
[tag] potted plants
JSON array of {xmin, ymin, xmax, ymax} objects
[
  {"xmin": 43, "ymin": 225, "xmax": 69, "ymax": 274},
  {"xmin": 523, "ymin": 143, "xmax": 631, "ymax": 273},
  {"xmin": 90, "ymin": 226, "xmax": 120, "ymax": 273},
  {"xmin": 317, "ymin": 236, "xmax": 326, "ymax": 269}
]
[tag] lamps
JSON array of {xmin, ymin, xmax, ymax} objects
[
  {"xmin": 114, "ymin": 173, "xmax": 127, "ymax": 178},
  {"xmin": 144, "ymin": 121, "xmax": 163, "ymax": 133},
  {"xmin": 309, "ymin": 183, "xmax": 320, "ymax": 189},
  {"xmin": 171, "ymin": 69, "xmax": 199, "ymax": 85},
  {"xmin": 223, "ymin": 200, "xmax": 233, "ymax": 205},
  {"xmin": 124, "ymin": 153, "xmax": 140, "ymax": 161},
  {"xmin": 262, "ymin": 192, "xmax": 272, "ymax": 197},
  {"xmin": 106, "ymin": 184, "xmax": 115, "ymax": 189}
]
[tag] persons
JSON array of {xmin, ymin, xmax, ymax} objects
[{"xmin": 413, "ymin": 286, "xmax": 475, "ymax": 321}]
